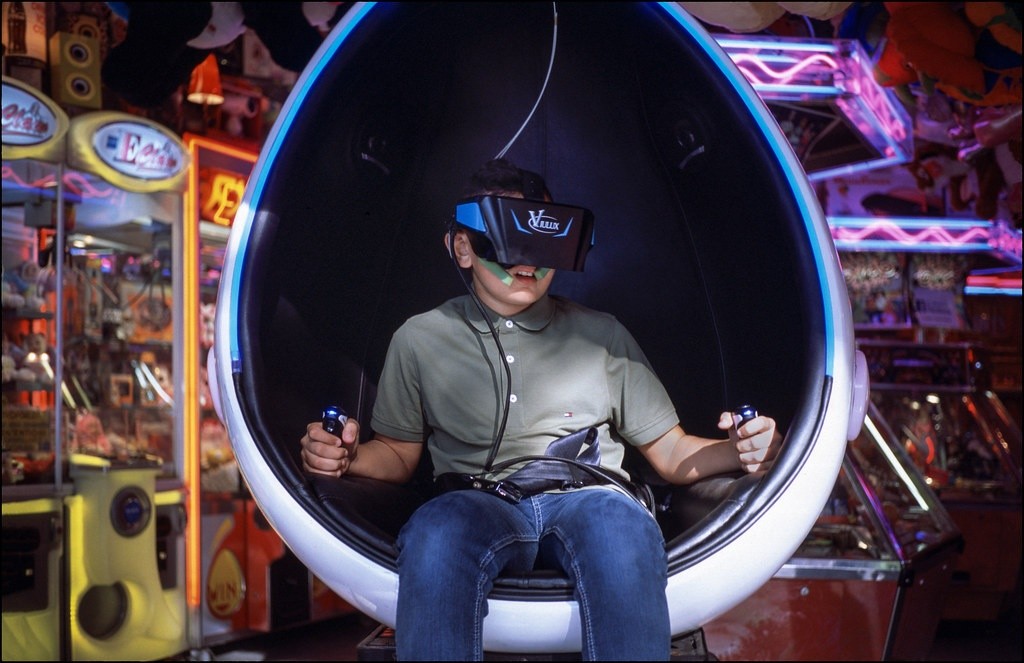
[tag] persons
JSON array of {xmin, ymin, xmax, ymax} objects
[{"xmin": 299, "ymin": 161, "xmax": 782, "ymax": 663}]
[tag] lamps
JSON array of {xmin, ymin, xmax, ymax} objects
[{"xmin": 187, "ymin": 53, "xmax": 225, "ymax": 105}]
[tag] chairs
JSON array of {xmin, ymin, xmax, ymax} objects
[{"xmin": 207, "ymin": 0, "xmax": 870, "ymax": 653}]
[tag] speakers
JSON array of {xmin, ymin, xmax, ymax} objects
[{"xmin": 51, "ymin": 32, "xmax": 103, "ymax": 111}]
